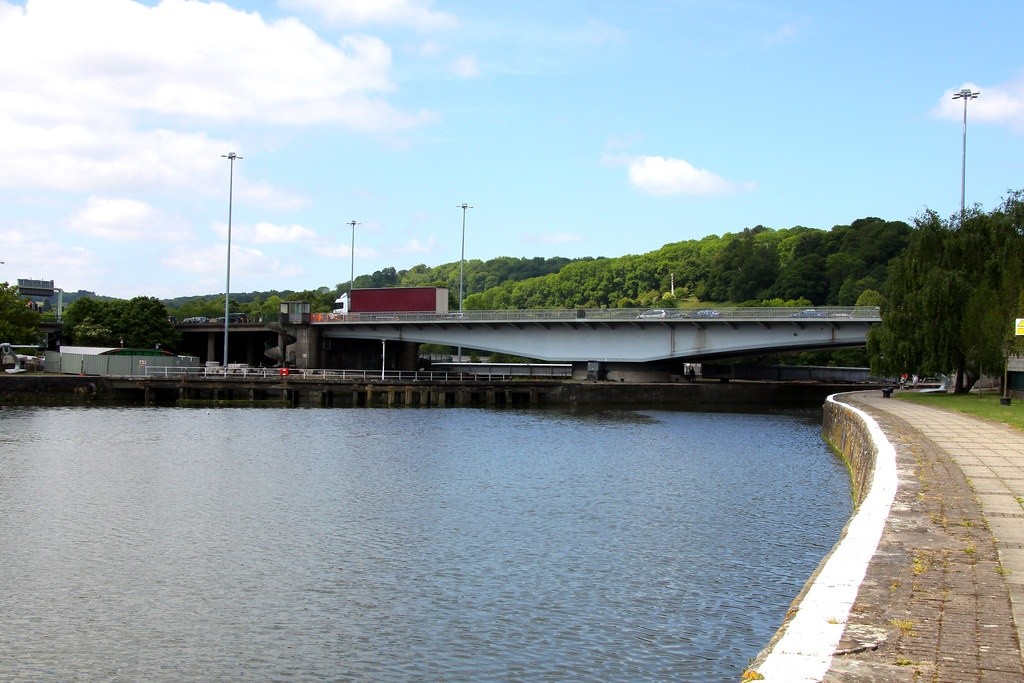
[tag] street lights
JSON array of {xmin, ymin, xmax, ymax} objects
[
  {"xmin": 456, "ymin": 203, "xmax": 474, "ymax": 307},
  {"xmin": 221, "ymin": 153, "xmax": 243, "ymax": 364},
  {"xmin": 346, "ymin": 220, "xmax": 361, "ymax": 287}
]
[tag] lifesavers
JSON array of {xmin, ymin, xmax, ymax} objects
[
  {"xmin": 87, "ymin": 383, "xmax": 96, "ymax": 400},
  {"xmin": 73, "ymin": 387, "xmax": 84, "ymax": 399}
]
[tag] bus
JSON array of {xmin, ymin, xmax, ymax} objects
[{"xmin": 376, "ymin": 317, "xmax": 399, "ymax": 321}]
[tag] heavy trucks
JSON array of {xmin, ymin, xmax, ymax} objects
[{"xmin": 333, "ymin": 287, "xmax": 449, "ymax": 318}]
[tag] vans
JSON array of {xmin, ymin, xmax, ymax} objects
[
  {"xmin": 635, "ymin": 309, "xmax": 671, "ymax": 319},
  {"xmin": 447, "ymin": 313, "xmax": 470, "ymax": 320}
]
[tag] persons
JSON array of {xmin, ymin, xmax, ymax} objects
[
  {"xmin": 690, "ymin": 367, "xmax": 695, "ymax": 382},
  {"xmin": 900, "ymin": 376, "xmax": 906, "ymax": 390},
  {"xmin": 912, "ymin": 374, "xmax": 918, "ymax": 389}
]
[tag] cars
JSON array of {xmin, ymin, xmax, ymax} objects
[
  {"xmin": 872, "ymin": 306, "xmax": 883, "ymax": 317},
  {"xmin": 672, "ymin": 309, "xmax": 724, "ymax": 318},
  {"xmin": 789, "ymin": 309, "xmax": 855, "ymax": 318},
  {"xmin": 165, "ymin": 312, "xmax": 250, "ymax": 323}
]
